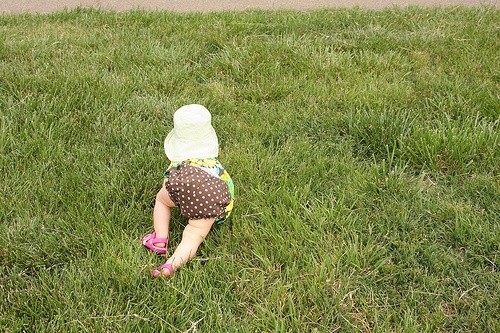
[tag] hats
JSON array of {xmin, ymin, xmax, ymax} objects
[{"xmin": 164, "ymin": 103, "xmax": 219, "ymax": 162}]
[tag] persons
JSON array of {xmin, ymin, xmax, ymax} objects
[{"xmin": 142, "ymin": 103, "xmax": 236, "ymax": 277}]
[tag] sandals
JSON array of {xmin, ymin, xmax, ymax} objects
[
  {"xmin": 142, "ymin": 230, "xmax": 169, "ymax": 254},
  {"xmin": 152, "ymin": 264, "xmax": 174, "ymax": 279}
]
